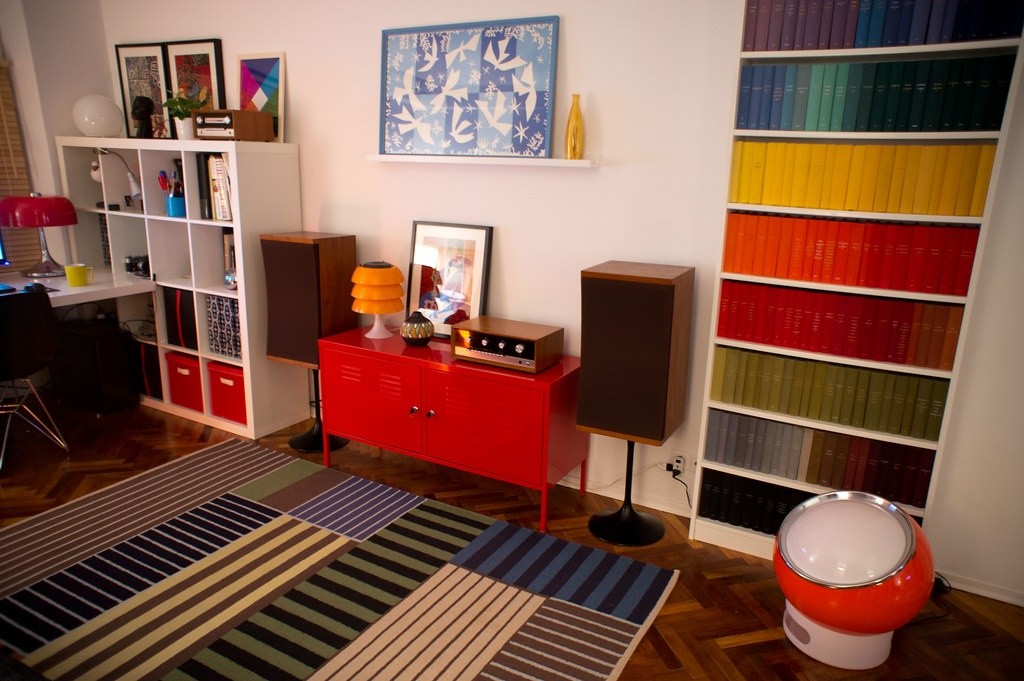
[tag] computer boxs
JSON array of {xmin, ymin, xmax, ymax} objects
[{"xmin": 48, "ymin": 318, "xmax": 134, "ymax": 413}]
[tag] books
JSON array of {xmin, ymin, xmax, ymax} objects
[
  {"xmin": 727, "ymin": 139, "xmax": 998, "ymax": 218},
  {"xmin": 194, "ymin": 152, "xmax": 234, "ymax": 222},
  {"xmin": 714, "ymin": 279, "xmax": 964, "ymax": 372},
  {"xmin": 703, "ymin": 407, "xmax": 937, "ymax": 509},
  {"xmin": 696, "ymin": 466, "xmax": 924, "ymax": 537},
  {"xmin": 720, "ymin": 211, "xmax": 980, "ymax": 297},
  {"xmin": 735, "ymin": 56, "xmax": 1019, "ymax": 133},
  {"xmin": 709, "ymin": 346, "xmax": 950, "ymax": 442},
  {"xmin": 222, "ymin": 226, "xmax": 237, "ymax": 292},
  {"xmin": 740, "ymin": 0, "xmax": 1024, "ymax": 53}
]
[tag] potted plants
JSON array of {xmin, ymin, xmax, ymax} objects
[{"xmin": 160, "ymin": 89, "xmax": 208, "ymax": 139}]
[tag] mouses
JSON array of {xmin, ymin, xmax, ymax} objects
[{"xmin": 24, "ymin": 283, "xmax": 45, "ymax": 292}]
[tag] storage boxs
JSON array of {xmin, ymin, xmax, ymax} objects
[
  {"xmin": 164, "ymin": 351, "xmax": 204, "ymax": 412},
  {"xmin": 205, "ymin": 360, "xmax": 249, "ymax": 425}
]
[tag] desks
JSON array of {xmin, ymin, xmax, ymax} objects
[{"xmin": 0, "ymin": 266, "xmax": 159, "ymax": 309}]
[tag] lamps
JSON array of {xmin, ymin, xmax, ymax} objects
[
  {"xmin": 75, "ymin": 144, "xmax": 142, "ymax": 200},
  {"xmin": 2, "ymin": 192, "xmax": 82, "ymax": 278},
  {"xmin": 352, "ymin": 262, "xmax": 405, "ymax": 341}
]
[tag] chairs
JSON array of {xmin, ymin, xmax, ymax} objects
[{"xmin": 0, "ymin": 291, "xmax": 70, "ymax": 472}]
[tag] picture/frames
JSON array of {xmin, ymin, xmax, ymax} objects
[
  {"xmin": 377, "ymin": 15, "xmax": 558, "ymax": 154},
  {"xmin": 237, "ymin": 52, "xmax": 287, "ymax": 143},
  {"xmin": 166, "ymin": 39, "xmax": 226, "ymax": 140},
  {"xmin": 406, "ymin": 220, "xmax": 493, "ymax": 341},
  {"xmin": 113, "ymin": 43, "xmax": 175, "ymax": 139}
]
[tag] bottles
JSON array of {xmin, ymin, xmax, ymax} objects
[
  {"xmin": 399, "ymin": 312, "xmax": 435, "ymax": 346},
  {"xmin": 565, "ymin": 95, "xmax": 586, "ymax": 160}
]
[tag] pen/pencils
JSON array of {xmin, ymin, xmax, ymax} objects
[{"xmin": 158, "ymin": 171, "xmax": 185, "ymax": 197}]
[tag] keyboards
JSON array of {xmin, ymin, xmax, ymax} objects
[{"xmin": 0, "ymin": 283, "xmax": 16, "ymax": 294}]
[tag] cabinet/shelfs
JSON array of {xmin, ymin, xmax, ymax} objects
[
  {"xmin": 318, "ymin": 324, "xmax": 586, "ymax": 535},
  {"xmin": 54, "ymin": 133, "xmax": 308, "ymax": 441},
  {"xmin": 686, "ymin": 0, "xmax": 1024, "ymax": 564}
]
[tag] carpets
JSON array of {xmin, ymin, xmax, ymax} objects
[{"xmin": 0, "ymin": 438, "xmax": 680, "ymax": 681}]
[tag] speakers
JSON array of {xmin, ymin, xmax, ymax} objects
[
  {"xmin": 260, "ymin": 231, "xmax": 357, "ymax": 370},
  {"xmin": 575, "ymin": 261, "xmax": 696, "ymax": 447}
]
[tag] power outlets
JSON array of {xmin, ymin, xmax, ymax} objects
[{"xmin": 672, "ymin": 452, "xmax": 690, "ymax": 477}]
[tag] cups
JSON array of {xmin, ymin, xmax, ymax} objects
[
  {"xmin": 64, "ymin": 263, "xmax": 93, "ymax": 288},
  {"xmin": 165, "ymin": 196, "xmax": 186, "ymax": 217}
]
